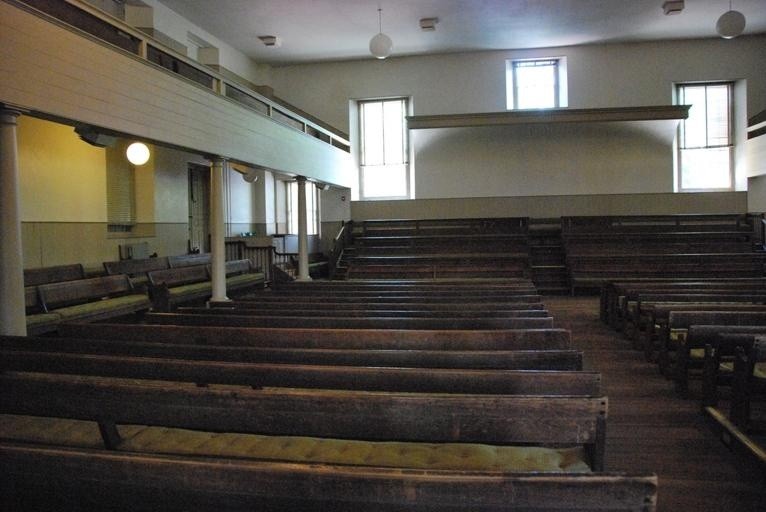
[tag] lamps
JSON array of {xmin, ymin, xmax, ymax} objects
[
  {"xmin": 715, "ymin": 0, "xmax": 746, "ymax": 39},
  {"xmin": 125, "ymin": 141, "xmax": 151, "ymax": 167},
  {"xmin": 369, "ymin": 8, "xmax": 393, "ymax": 60}
]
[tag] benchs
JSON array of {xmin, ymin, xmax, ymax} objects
[{"xmin": 0, "ymin": 211, "xmax": 766, "ymax": 512}]
